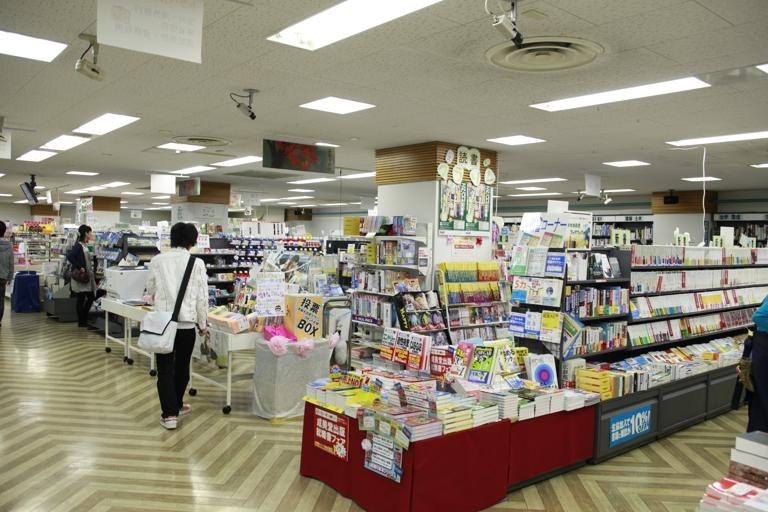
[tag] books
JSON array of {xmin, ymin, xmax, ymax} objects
[
  {"xmin": 302, "ymin": 216, "xmax": 768, "ymax": 441},
  {"xmin": 699, "ymin": 432, "xmax": 768, "ymax": 510}
]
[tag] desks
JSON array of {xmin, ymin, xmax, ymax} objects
[
  {"xmin": 99, "ymin": 289, "xmax": 265, "ymax": 418},
  {"xmin": 302, "ymin": 404, "xmax": 594, "ymax": 512}
]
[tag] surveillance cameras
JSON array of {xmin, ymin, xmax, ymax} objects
[
  {"xmin": 301, "ymin": 208, "xmax": 305, "ymax": 215},
  {"xmin": 230, "ymin": 89, "xmax": 259, "ymax": 120},
  {"xmin": 577, "ymin": 190, "xmax": 584, "ymax": 201},
  {"xmin": 484, "ymin": 0, "xmax": 523, "ymax": 49}
]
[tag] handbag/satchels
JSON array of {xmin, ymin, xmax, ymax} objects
[
  {"xmin": 72, "ymin": 268, "xmax": 90, "ymax": 284},
  {"xmin": 137, "ymin": 310, "xmax": 178, "ymax": 355}
]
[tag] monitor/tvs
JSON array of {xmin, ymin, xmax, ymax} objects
[{"xmin": 19, "ymin": 182, "xmax": 39, "ymax": 205}]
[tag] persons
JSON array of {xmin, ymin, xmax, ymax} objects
[
  {"xmin": 66, "ymin": 224, "xmax": 97, "ymax": 328},
  {"xmin": 0, "ymin": 220, "xmax": 14, "ymax": 328},
  {"xmin": 145, "ymin": 223, "xmax": 209, "ymax": 428}
]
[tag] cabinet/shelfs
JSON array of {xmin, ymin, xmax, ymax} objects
[
  {"xmin": 344, "ymin": 209, "xmax": 413, "ymax": 350},
  {"xmin": 566, "ymin": 250, "xmax": 628, "ymax": 353},
  {"xmin": 198, "ymin": 240, "xmax": 318, "ymax": 300},
  {"xmin": 634, "ymin": 259, "xmax": 708, "ymax": 346},
  {"xmin": 441, "ymin": 262, "xmax": 510, "ymax": 339},
  {"xmin": 94, "ymin": 232, "xmax": 127, "ymax": 336}
]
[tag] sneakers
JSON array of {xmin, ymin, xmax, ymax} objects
[
  {"xmin": 178, "ymin": 404, "xmax": 191, "ymax": 415},
  {"xmin": 160, "ymin": 415, "xmax": 178, "ymax": 428}
]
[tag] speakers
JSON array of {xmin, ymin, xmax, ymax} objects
[{"xmin": 664, "ymin": 195, "xmax": 679, "ymax": 204}]
